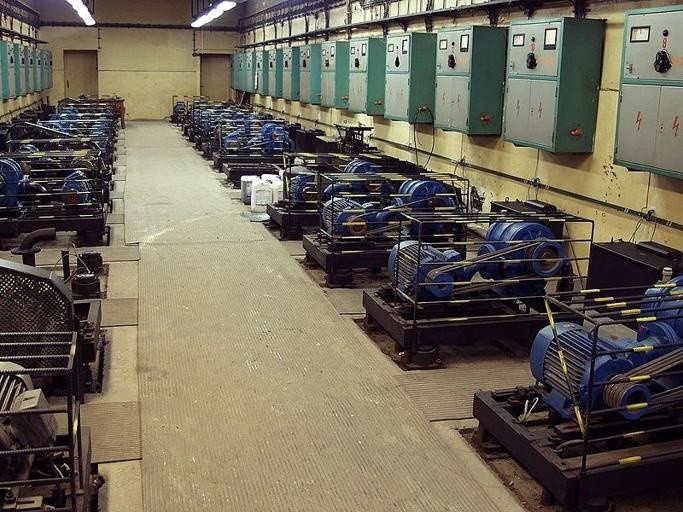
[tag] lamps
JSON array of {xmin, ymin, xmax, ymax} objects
[
  {"xmin": 190, "ymin": 0, "xmax": 237, "ymax": 28},
  {"xmin": 62, "ymin": 0, "xmax": 96, "ymax": 28}
]
